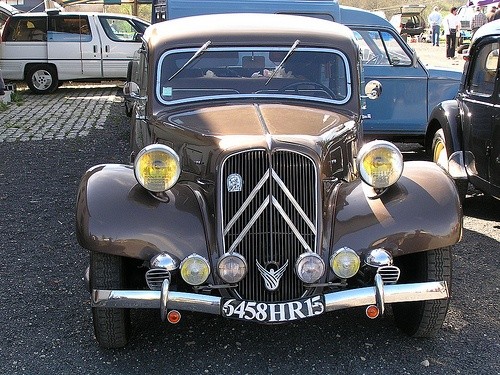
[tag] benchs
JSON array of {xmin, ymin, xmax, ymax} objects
[{"xmin": 164, "ymin": 78, "xmax": 315, "ymax": 94}]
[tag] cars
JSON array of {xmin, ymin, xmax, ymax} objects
[
  {"xmin": 426, "ymin": 18, "xmax": 500, "ymax": 207},
  {"xmin": 75, "ymin": 13, "xmax": 464, "ymax": 352},
  {"xmin": 457, "ymin": 32, "xmax": 474, "ymax": 54}
]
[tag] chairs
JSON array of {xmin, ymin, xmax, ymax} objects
[{"xmin": 179, "ymin": 66, "xmax": 288, "ymax": 79}]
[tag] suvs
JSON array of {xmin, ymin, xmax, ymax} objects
[{"xmin": 419, "ymin": 28, "xmax": 442, "ymax": 43}]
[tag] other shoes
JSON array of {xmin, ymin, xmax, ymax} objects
[
  {"xmin": 448, "ymin": 57, "xmax": 450, "ymax": 59},
  {"xmin": 451, "ymin": 56, "xmax": 454, "ymax": 58},
  {"xmin": 433, "ymin": 44, "xmax": 435, "ymax": 46},
  {"xmin": 436, "ymin": 45, "xmax": 439, "ymax": 46}
]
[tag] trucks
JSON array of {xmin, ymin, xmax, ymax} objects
[{"xmin": 166, "ymin": 0, "xmax": 463, "ymax": 149}]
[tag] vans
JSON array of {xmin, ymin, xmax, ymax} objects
[
  {"xmin": 0, "ymin": 1, "xmax": 152, "ymax": 95},
  {"xmin": 390, "ymin": 5, "xmax": 427, "ymax": 37}
]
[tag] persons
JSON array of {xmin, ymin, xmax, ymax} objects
[
  {"xmin": 443, "ymin": 6, "xmax": 460, "ymax": 58},
  {"xmin": 469, "ymin": 5, "xmax": 486, "ymax": 39},
  {"xmin": 427, "ymin": 6, "xmax": 440, "ymax": 46},
  {"xmin": 486, "ymin": 2, "xmax": 500, "ymax": 21}
]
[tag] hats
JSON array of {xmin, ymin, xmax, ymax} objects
[{"xmin": 476, "ymin": 6, "xmax": 481, "ymax": 11}]
